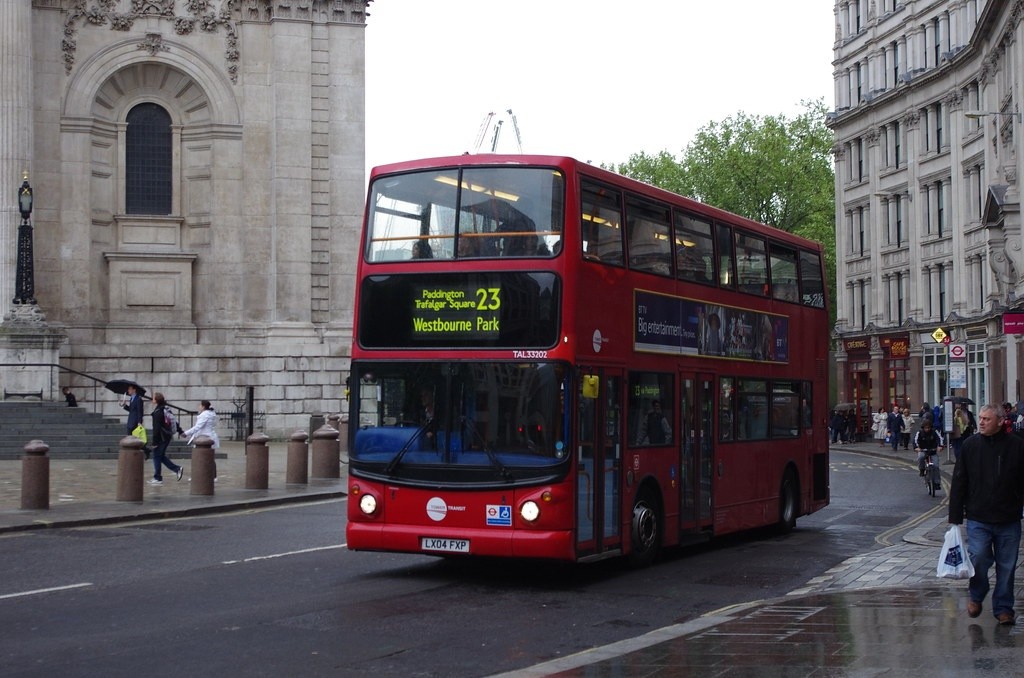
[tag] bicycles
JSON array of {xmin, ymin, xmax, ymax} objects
[{"xmin": 914, "ymin": 448, "xmax": 944, "ymax": 498}]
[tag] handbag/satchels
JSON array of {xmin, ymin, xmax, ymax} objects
[
  {"xmin": 951, "ymin": 418, "xmax": 961, "ymax": 439},
  {"xmin": 936, "ymin": 524, "xmax": 975, "ymax": 580},
  {"xmin": 131, "ymin": 426, "xmax": 147, "ymax": 445},
  {"xmin": 871, "ymin": 423, "xmax": 879, "ymax": 431}
]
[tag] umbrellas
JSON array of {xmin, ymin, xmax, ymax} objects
[
  {"xmin": 833, "ymin": 403, "xmax": 857, "ymax": 415},
  {"xmin": 105, "ymin": 379, "xmax": 147, "ymax": 407},
  {"xmin": 944, "ymin": 395, "xmax": 976, "ymax": 412}
]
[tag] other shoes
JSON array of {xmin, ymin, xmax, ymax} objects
[
  {"xmin": 147, "ymin": 478, "xmax": 162, "ymax": 484},
  {"xmin": 188, "ymin": 477, "xmax": 192, "ymax": 481},
  {"xmin": 177, "ymin": 467, "xmax": 183, "ymax": 481},
  {"xmin": 214, "ymin": 478, "xmax": 217, "ymax": 482},
  {"xmin": 145, "ymin": 448, "xmax": 151, "ymax": 461},
  {"xmin": 920, "ymin": 470, "xmax": 924, "ymax": 476},
  {"xmin": 904, "ymin": 447, "xmax": 908, "ymax": 450},
  {"xmin": 936, "ymin": 484, "xmax": 941, "ymax": 489}
]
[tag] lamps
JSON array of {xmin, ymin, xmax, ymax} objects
[
  {"xmin": 964, "ymin": 110, "xmax": 1023, "ymax": 125},
  {"xmin": 874, "ymin": 190, "xmax": 912, "ymax": 201}
]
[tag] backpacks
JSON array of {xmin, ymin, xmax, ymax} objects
[{"xmin": 155, "ymin": 407, "xmax": 177, "ymax": 435}]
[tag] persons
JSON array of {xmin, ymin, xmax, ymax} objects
[
  {"xmin": 62, "ymin": 387, "xmax": 78, "ymax": 407},
  {"xmin": 123, "ymin": 386, "xmax": 143, "ymax": 435},
  {"xmin": 947, "ymin": 403, "xmax": 1024, "ymax": 625},
  {"xmin": 636, "ymin": 400, "xmax": 673, "ymax": 444},
  {"xmin": 830, "ymin": 402, "xmax": 1024, "ymax": 476},
  {"xmin": 146, "ymin": 392, "xmax": 187, "ymax": 484},
  {"xmin": 402, "ymin": 386, "xmax": 451, "ymax": 437},
  {"xmin": 410, "ymin": 242, "xmax": 433, "ymax": 260},
  {"xmin": 181, "ymin": 400, "xmax": 219, "ymax": 483},
  {"xmin": 912, "ymin": 418, "xmax": 944, "ymax": 490},
  {"xmin": 491, "ymin": 196, "xmax": 538, "ymax": 256}
]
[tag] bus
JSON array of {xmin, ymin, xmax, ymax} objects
[{"xmin": 346, "ymin": 153, "xmax": 829, "ymax": 574}]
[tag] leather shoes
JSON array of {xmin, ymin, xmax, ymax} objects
[
  {"xmin": 968, "ymin": 599, "xmax": 982, "ymax": 618},
  {"xmin": 999, "ymin": 612, "xmax": 1015, "ymax": 625}
]
